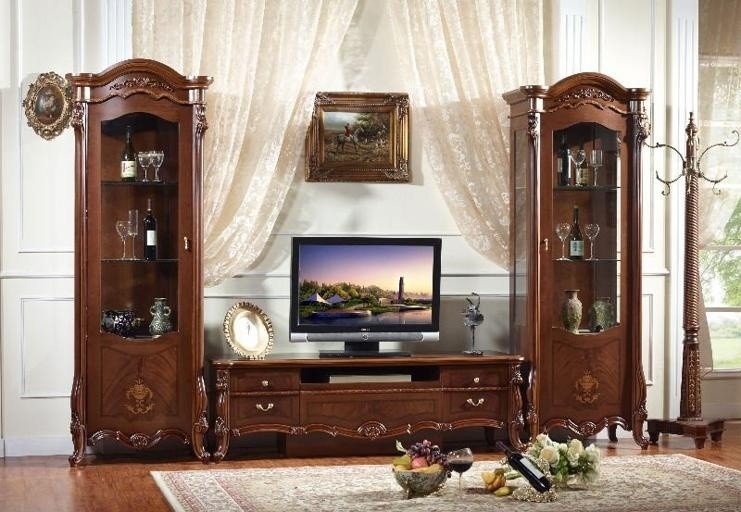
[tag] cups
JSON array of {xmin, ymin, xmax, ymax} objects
[
  {"xmin": 116, "ymin": 221, "xmax": 131, "ymax": 260},
  {"xmin": 138, "ymin": 152, "xmax": 151, "ymax": 182},
  {"xmin": 448, "ymin": 447, "xmax": 474, "ymax": 497},
  {"xmin": 588, "ymin": 150, "xmax": 603, "ymax": 186},
  {"xmin": 571, "ymin": 150, "xmax": 587, "ymax": 187},
  {"xmin": 556, "ymin": 223, "xmax": 572, "ymax": 260},
  {"xmin": 150, "ymin": 151, "xmax": 164, "ymax": 182},
  {"xmin": 584, "ymin": 223, "xmax": 601, "ymax": 260},
  {"xmin": 128, "ymin": 210, "xmax": 139, "ymax": 260}
]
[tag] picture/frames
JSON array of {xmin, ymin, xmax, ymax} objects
[
  {"xmin": 24, "ymin": 71, "xmax": 73, "ymax": 141},
  {"xmin": 302, "ymin": 91, "xmax": 413, "ymax": 185},
  {"xmin": 223, "ymin": 301, "xmax": 275, "ymax": 361}
]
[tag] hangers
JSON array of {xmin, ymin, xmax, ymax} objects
[{"xmin": 645, "ymin": 108, "xmax": 740, "ymax": 452}]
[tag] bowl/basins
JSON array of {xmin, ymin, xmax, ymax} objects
[{"xmin": 392, "ymin": 467, "xmax": 448, "ymax": 499}]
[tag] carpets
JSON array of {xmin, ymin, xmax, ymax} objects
[{"xmin": 150, "ymin": 452, "xmax": 741, "ymax": 511}]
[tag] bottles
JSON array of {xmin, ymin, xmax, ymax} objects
[
  {"xmin": 120, "ymin": 126, "xmax": 138, "ymax": 182},
  {"xmin": 496, "ymin": 440, "xmax": 552, "ymax": 493},
  {"xmin": 557, "ymin": 133, "xmax": 571, "ymax": 186},
  {"xmin": 567, "ymin": 206, "xmax": 585, "ymax": 261},
  {"xmin": 577, "ymin": 139, "xmax": 590, "ymax": 187},
  {"xmin": 143, "ymin": 199, "xmax": 158, "ymax": 260}
]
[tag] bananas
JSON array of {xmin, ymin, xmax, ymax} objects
[{"xmin": 481, "ymin": 468, "xmax": 506, "ymax": 492}]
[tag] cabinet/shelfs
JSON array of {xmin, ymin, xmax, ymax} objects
[
  {"xmin": 65, "ymin": 57, "xmax": 213, "ymax": 467},
  {"xmin": 501, "ymin": 72, "xmax": 653, "ymax": 450},
  {"xmin": 208, "ymin": 349, "xmax": 536, "ymax": 462}
]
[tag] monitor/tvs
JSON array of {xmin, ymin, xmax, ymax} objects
[{"xmin": 290, "ymin": 237, "xmax": 441, "ymax": 358}]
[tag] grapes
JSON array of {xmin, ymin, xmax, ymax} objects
[{"xmin": 406, "ymin": 439, "xmax": 453, "ymax": 479}]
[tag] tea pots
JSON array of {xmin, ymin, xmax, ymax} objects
[{"xmin": 102, "ymin": 309, "xmax": 146, "ymax": 337}]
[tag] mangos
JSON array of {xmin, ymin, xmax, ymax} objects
[{"xmin": 494, "ymin": 487, "xmax": 510, "ymax": 497}]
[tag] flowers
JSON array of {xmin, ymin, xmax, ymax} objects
[{"xmin": 526, "ymin": 432, "xmax": 601, "ymax": 487}]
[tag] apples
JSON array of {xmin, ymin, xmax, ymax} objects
[{"xmin": 411, "ymin": 457, "xmax": 428, "ymax": 469}]
